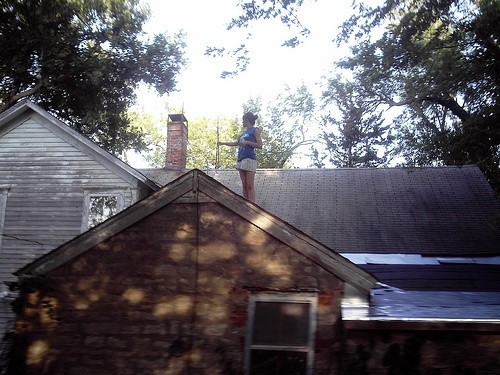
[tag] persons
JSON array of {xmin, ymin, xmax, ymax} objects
[{"xmin": 217, "ymin": 111, "xmax": 263, "ymax": 203}]
